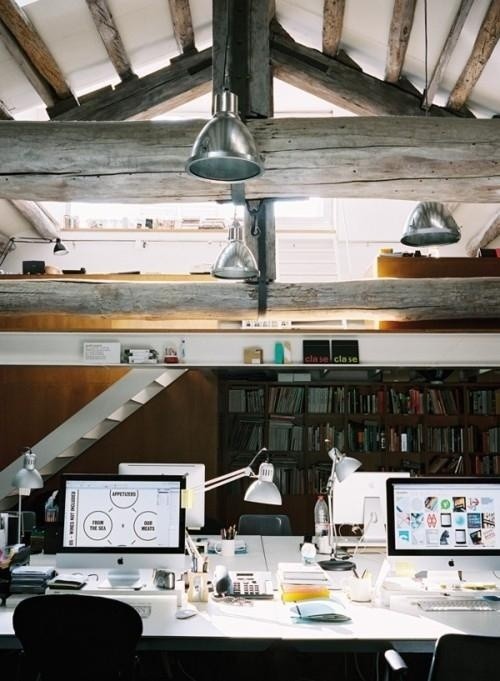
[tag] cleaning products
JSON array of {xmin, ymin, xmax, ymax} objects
[
  {"xmin": 178, "ymin": 340, "xmax": 189, "ymax": 363},
  {"xmin": 283, "ymin": 340, "xmax": 292, "ymax": 364}
]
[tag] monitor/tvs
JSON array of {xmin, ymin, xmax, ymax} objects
[
  {"xmin": 385, "ymin": 476, "xmax": 500, "ymax": 591},
  {"xmin": 333, "ymin": 471, "xmax": 411, "ymax": 546},
  {"xmin": 55, "ymin": 474, "xmax": 186, "ymax": 589},
  {"xmin": 118, "ymin": 462, "xmax": 206, "ymax": 537}
]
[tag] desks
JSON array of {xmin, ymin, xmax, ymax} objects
[{"xmin": 0, "ymin": 528, "xmax": 500, "ymax": 652}]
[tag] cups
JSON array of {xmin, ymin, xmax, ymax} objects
[
  {"xmin": 164, "ymin": 355, "xmax": 179, "ymax": 363},
  {"xmin": 187, "ymin": 571, "xmax": 209, "ymax": 602},
  {"xmin": 214, "ymin": 540, "xmax": 236, "ymax": 557},
  {"xmin": 346, "ymin": 574, "xmax": 373, "ymax": 602}
]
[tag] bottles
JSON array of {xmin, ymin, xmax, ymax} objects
[
  {"xmin": 274, "ymin": 341, "xmax": 292, "ymax": 364},
  {"xmin": 315, "ymin": 495, "xmax": 329, "ymax": 536},
  {"xmin": 180, "ymin": 339, "xmax": 186, "ymax": 363}
]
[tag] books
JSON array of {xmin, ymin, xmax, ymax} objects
[
  {"xmin": 278, "ymin": 562, "xmax": 352, "ymax": 623},
  {"xmin": 226, "ymin": 384, "xmax": 499, "ymax": 497},
  {"xmin": 122, "ymin": 346, "xmax": 161, "ymax": 366}
]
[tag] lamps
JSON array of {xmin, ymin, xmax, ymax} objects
[
  {"xmin": 12, "ymin": 446, "xmax": 46, "ymax": 546},
  {"xmin": 399, "ymin": 203, "xmax": 462, "ymax": 249},
  {"xmin": 209, "ymin": 204, "xmax": 265, "ymax": 282},
  {"xmin": 315, "ymin": 445, "xmax": 365, "ymax": 572},
  {"xmin": 0, "ymin": 233, "xmax": 68, "ymax": 273},
  {"xmin": 181, "ymin": 447, "xmax": 287, "ymax": 591},
  {"xmin": 178, "ymin": 0, "xmax": 267, "ymax": 185}
]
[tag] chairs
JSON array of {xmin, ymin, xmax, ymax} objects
[
  {"xmin": 13, "ymin": 594, "xmax": 144, "ymax": 679},
  {"xmin": 342, "ymin": 633, "xmax": 500, "ymax": 681}
]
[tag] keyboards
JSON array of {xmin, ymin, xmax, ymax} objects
[{"xmin": 416, "ymin": 598, "xmax": 492, "ymax": 612}]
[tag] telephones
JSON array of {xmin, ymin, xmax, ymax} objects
[{"xmin": 215, "ymin": 566, "xmax": 274, "ymax": 600}]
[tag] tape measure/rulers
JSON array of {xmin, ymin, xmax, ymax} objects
[{"xmin": 185, "ymin": 531, "xmax": 203, "ymax": 573}]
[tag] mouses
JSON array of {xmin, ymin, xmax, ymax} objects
[{"xmin": 175, "ymin": 606, "xmax": 196, "ymax": 619}]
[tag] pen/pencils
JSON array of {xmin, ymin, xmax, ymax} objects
[
  {"xmin": 352, "ymin": 567, "xmax": 368, "ymax": 579},
  {"xmin": 203, "ymin": 561, "xmax": 209, "ymax": 573},
  {"xmin": 221, "ymin": 523, "xmax": 238, "ymax": 541},
  {"xmin": 192, "ymin": 555, "xmax": 198, "ymax": 574}
]
